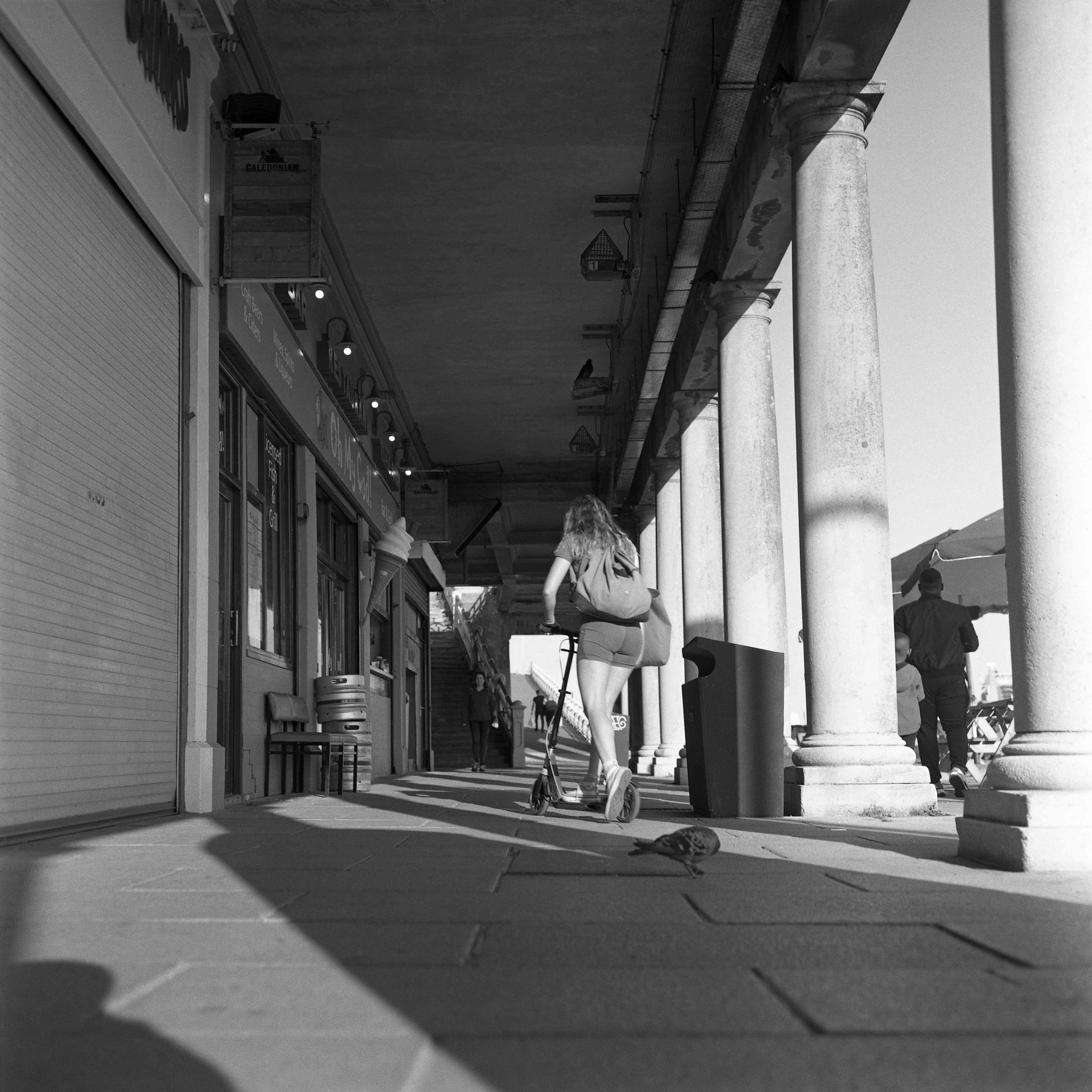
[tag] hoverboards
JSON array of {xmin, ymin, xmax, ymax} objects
[{"xmin": 526, "ymin": 621, "xmax": 641, "ymax": 824}]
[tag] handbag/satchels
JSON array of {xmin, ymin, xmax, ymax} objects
[
  {"xmin": 527, "ymin": 715, "xmax": 535, "ymax": 727},
  {"xmin": 638, "ymin": 588, "xmax": 672, "ymax": 667},
  {"xmin": 559, "ymin": 717, "xmax": 563, "ymax": 727}
]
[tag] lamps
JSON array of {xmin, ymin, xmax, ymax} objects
[
  {"xmin": 393, "ymin": 447, "xmax": 416, "ymax": 476},
  {"xmin": 357, "ymin": 375, "xmax": 384, "ymax": 408},
  {"xmin": 305, "ymin": 283, "xmax": 332, "ymax": 299},
  {"xmin": 322, "ymin": 317, "xmax": 357, "ymax": 355},
  {"xmin": 372, "ymin": 411, "xmax": 402, "ymax": 442}
]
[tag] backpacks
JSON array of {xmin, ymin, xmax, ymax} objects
[{"xmin": 568, "ymin": 543, "xmax": 652, "ymax": 622}]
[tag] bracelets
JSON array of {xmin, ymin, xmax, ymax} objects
[{"xmin": 543, "ymin": 616, "xmax": 555, "ymax": 625}]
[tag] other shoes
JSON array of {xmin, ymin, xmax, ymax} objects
[
  {"xmin": 479, "ymin": 765, "xmax": 486, "ymax": 773},
  {"xmin": 541, "ymin": 728, "xmax": 545, "ymax": 733},
  {"xmin": 471, "ymin": 763, "xmax": 478, "ymax": 772},
  {"xmin": 934, "ymin": 782, "xmax": 946, "ymax": 797},
  {"xmin": 533, "ymin": 728, "xmax": 539, "ymax": 733},
  {"xmin": 949, "ymin": 767, "xmax": 969, "ymax": 798}
]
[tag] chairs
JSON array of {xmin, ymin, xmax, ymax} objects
[{"xmin": 264, "ymin": 691, "xmax": 359, "ymax": 798}]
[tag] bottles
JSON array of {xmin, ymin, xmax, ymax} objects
[{"xmin": 377, "ymin": 657, "xmax": 389, "ymax": 673}]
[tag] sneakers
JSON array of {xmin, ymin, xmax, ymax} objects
[
  {"xmin": 605, "ymin": 767, "xmax": 633, "ymax": 821},
  {"xmin": 562, "ymin": 777, "xmax": 602, "ymax": 805}
]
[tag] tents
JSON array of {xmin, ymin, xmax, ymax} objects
[{"xmin": 893, "ymin": 508, "xmax": 1009, "ymax": 620}]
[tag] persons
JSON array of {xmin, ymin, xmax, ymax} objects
[
  {"xmin": 894, "ymin": 568, "xmax": 979, "ymax": 798},
  {"xmin": 531, "ymin": 690, "xmax": 557, "ymax": 733},
  {"xmin": 894, "ymin": 631, "xmax": 925, "ymax": 751},
  {"xmin": 541, "ymin": 493, "xmax": 644, "ymax": 822},
  {"xmin": 462, "ymin": 671, "xmax": 499, "ymax": 771}
]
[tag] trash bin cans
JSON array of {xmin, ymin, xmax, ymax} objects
[{"xmin": 682, "ymin": 635, "xmax": 786, "ymax": 819}]
